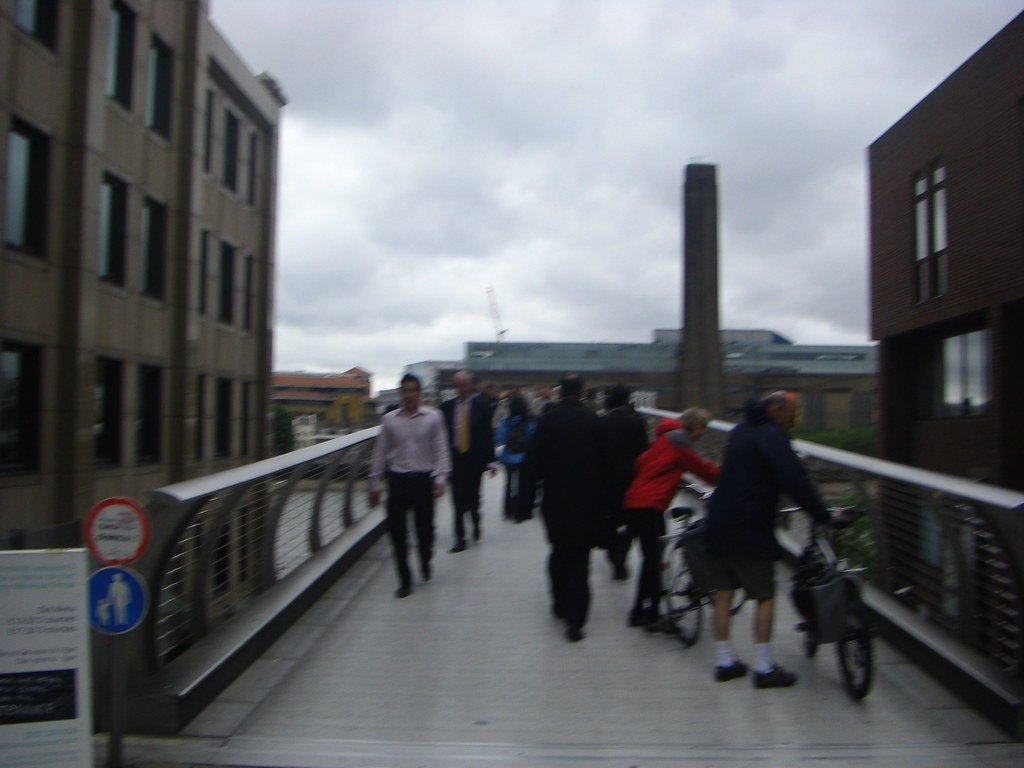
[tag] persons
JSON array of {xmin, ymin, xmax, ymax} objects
[{"xmin": 365, "ymin": 367, "xmax": 842, "ymax": 689}]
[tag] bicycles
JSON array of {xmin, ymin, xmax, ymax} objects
[
  {"xmin": 777, "ymin": 505, "xmax": 880, "ymax": 699},
  {"xmin": 659, "ymin": 486, "xmax": 716, "ymax": 647}
]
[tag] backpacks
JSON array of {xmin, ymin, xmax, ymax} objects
[{"xmin": 507, "ymin": 419, "xmax": 527, "ymax": 454}]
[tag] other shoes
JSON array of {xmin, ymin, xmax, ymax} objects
[
  {"xmin": 716, "ymin": 661, "xmax": 747, "ymax": 682},
  {"xmin": 629, "ymin": 609, "xmax": 657, "ymax": 625},
  {"xmin": 421, "ymin": 564, "xmax": 430, "ymax": 580},
  {"xmin": 754, "ymin": 663, "xmax": 800, "ymax": 687},
  {"xmin": 645, "ymin": 617, "xmax": 683, "ymax": 634},
  {"xmin": 563, "ymin": 617, "xmax": 585, "ymax": 642},
  {"xmin": 451, "ymin": 539, "xmax": 466, "ymax": 552},
  {"xmin": 398, "ymin": 584, "xmax": 410, "ymax": 598},
  {"xmin": 474, "ymin": 526, "xmax": 481, "ymax": 541}
]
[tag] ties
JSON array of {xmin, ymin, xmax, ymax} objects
[{"xmin": 460, "ymin": 402, "xmax": 468, "ymax": 453}]
